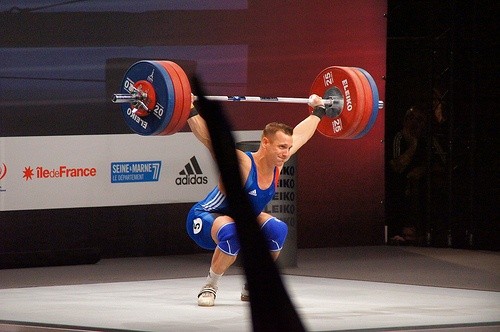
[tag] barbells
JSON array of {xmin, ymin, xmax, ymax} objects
[{"xmin": 112, "ymin": 57, "xmax": 384, "ymax": 140}]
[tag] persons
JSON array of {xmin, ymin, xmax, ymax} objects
[
  {"xmin": 187, "ymin": 93, "xmax": 326, "ymax": 306},
  {"xmin": 390, "ymin": 107, "xmax": 429, "ymax": 240}
]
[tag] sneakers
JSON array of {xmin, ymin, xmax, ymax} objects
[
  {"xmin": 197, "ymin": 284, "xmax": 218, "ymax": 306},
  {"xmin": 241, "ymin": 285, "xmax": 251, "ymax": 301}
]
